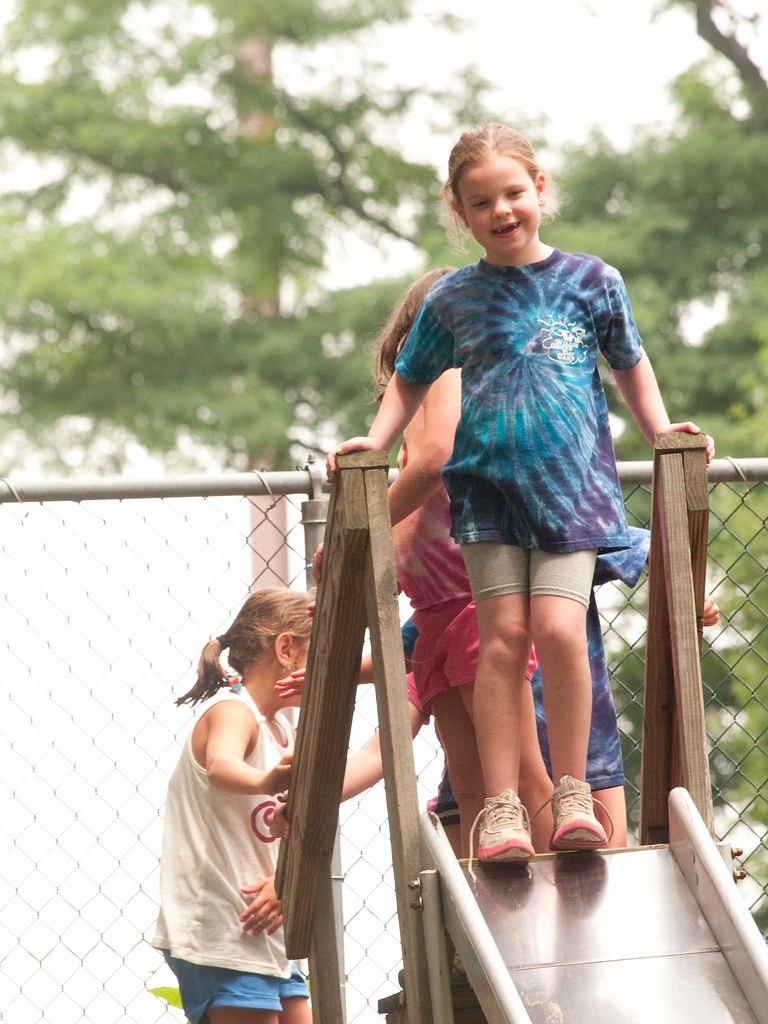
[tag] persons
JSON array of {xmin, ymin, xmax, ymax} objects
[
  {"xmin": 328, "ymin": 122, "xmax": 715, "ymax": 865},
  {"xmin": 268, "ymin": 265, "xmax": 722, "ymax": 987},
  {"xmin": 143, "ymin": 586, "xmax": 319, "ymax": 1024}
]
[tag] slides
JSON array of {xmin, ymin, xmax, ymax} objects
[{"xmin": 419, "ymin": 788, "xmax": 768, "ymax": 1024}]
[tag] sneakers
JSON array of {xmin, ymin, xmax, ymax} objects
[
  {"xmin": 549, "ymin": 775, "xmax": 610, "ymax": 852},
  {"xmin": 475, "ymin": 791, "xmax": 537, "ymax": 865}
]
